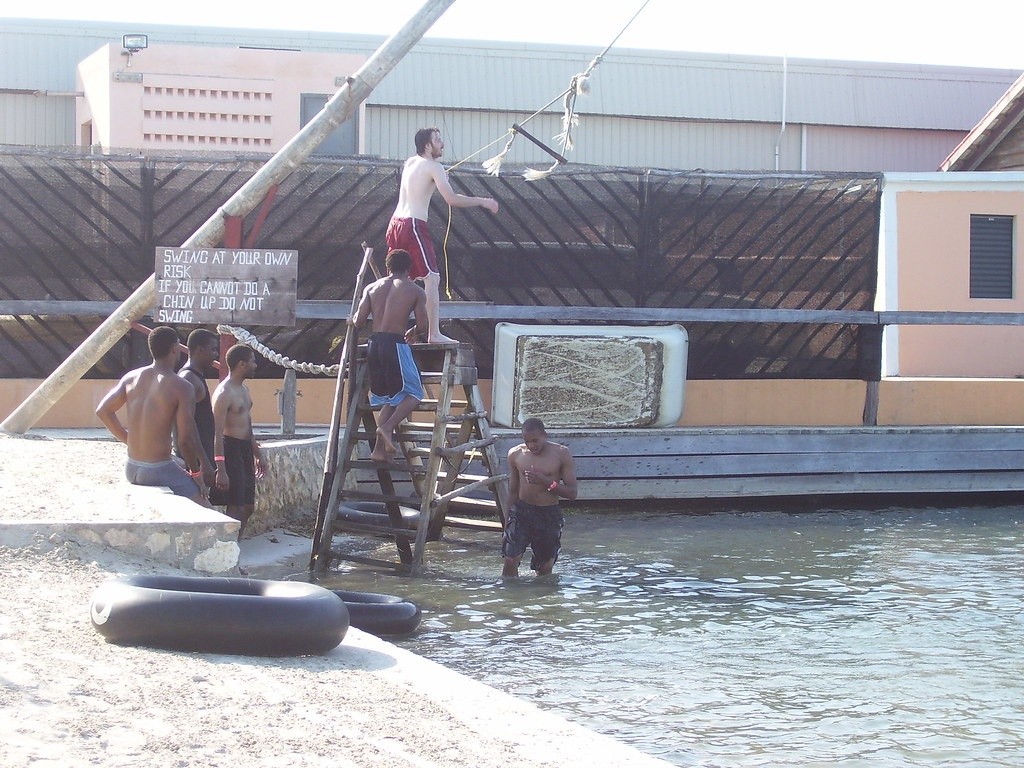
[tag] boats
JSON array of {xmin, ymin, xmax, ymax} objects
[{"xmin": 0, "ymin": 17, "xmax": 1024, "ymax": 515}]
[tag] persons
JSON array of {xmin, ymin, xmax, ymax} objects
[
  {"xmin": 502, "ymin": 417, "xmax": 578, "ymax": 577},
  {"xmin": 96, "ymin": 326, "xmax": 267, "ymax": 576},
  {"xmin": 345, "ymin": 249, "xmax": 428, "ymax": 465},
  {"xmin": 386, "ymin": 128, "xmax": 498, "ymax": 343}
]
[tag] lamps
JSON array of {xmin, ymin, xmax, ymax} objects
[{"xmin": 119, "ymin": 34, "xmax": 148, "ymax": 58}]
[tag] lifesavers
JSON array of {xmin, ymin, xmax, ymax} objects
[
  {"xmin": 88, "ymin": 574, "xmax": 355, "ymax": 656},
  {"xmin": 332, "ymin": 587, "xmax": 423, "ymax": 634},
  {"xmin": 335, "ymin": 501, "xmax": 421, "ymax": 533}
]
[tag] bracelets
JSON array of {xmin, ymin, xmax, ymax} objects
[
  {"xmin": 215, "ymin": 456, "xmax": 225, "ymax": 461},
  {"xmin": 191, "ymin": 471, "xmax": 201, "ymax": 477},
  {"xmin": 547, "ymin": 480, "xmax": 558, "ymax": 492}
]
